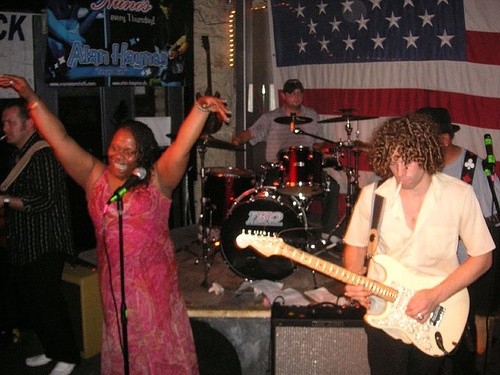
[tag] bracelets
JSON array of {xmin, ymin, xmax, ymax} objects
[
  {"xmin": 25, "ymin": 98, "xmax": 41, "ymax": 110},
  {"xmin": 196, "ymin": 102, "xmax": 210, "ymax": 112}
]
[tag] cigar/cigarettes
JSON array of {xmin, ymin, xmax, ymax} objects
[
  {"xmin": 0, "ymin": 134, "xmax": 8, "ymax": 140},
  {"xmin": 396, "ymin": 181, "xmax": 403, "ymax": 195}
]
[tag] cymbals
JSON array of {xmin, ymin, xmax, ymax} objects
[
  {"xmin": 338, "ymin": 108, "xmax": 357, "ymax": 112},
  {"xmin": 274, "ymin": 115, "xmax": 313, "ymax": 124},
  {"xmin": 165, "ymin": 132, "xmax": 245, "ymax": 153},
  {"xmin": 332, "ymin": 143, "xmax": 367, "ymax": 152},
  {"xmin": 317, "ymin": 113, "xmax": 379, "ymax": 127}
]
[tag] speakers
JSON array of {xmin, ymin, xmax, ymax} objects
[{"xmin": 270, "ymin": 301, "xmax": 372, "ymax": 375}]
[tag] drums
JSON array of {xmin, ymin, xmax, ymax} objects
[
  {"xmin": 320, "ymin": 147, "xmax": 341, "ymax": 168},
  {"xmin": 201, "ymin": 165, "xmax": 256, "ymax": 228},
  {"xmin": 219, "ymin": 187, "xmax": 307, "ymax": 281},
  {"xmin": 260, "ymin": 163, "xmax": 281, "ymax": 190},
  {"xmin": 276, "ymin": 146, "xmax": 324, "ymax": 196}
]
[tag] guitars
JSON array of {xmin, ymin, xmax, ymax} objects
[
  {"xmin": 196, "ymin": 35, "xmax": 227, "ymax": 135},
  {"xmin": 236, "ymin": 229, "xmax": 469, "ymax": 357},
  {"xmin": 485, "ymin": 213, "xmax": 500, "ymax": 227}
]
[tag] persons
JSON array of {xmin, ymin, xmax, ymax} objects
[
  {"xmin": 231, "ymin": 79, "xmax": 342, "ymax": 245},
  {"xmin": 0, "ymin": 73, "xmax": 230, "ymax": 375},
  {"xmin": 417, "ymin": 107, "xmax": 497, "ymax": 265},
  {"xmin": 42, "ymin": 0, "xmax": 189, "ymax": 78},
  {"xmin": 0, "ymin": 99, "xmax": 82, "ymax": 375},
  {"xmin": 342, "ymin": 115, "xmax": 496, "ymax": 375}
]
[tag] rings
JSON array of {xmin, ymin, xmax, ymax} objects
[{"xmin": 418, "ymin": 313, "xmax": 424, "ymax": 320}]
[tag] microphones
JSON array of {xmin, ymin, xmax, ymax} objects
[
  {"xmin": 105, "ymin": 167, "xmax": 148, "ymax": 205},
  {"xmin": 484, "ymin": 134, "xmax": 495, "ymax": 174}
]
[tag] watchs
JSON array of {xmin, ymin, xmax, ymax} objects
[{"xmin": 2, "ymin": 195, "xmax": 11, "ymax": 208}]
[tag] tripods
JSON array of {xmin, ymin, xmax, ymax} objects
[{"xmin": 174, "ymin": 147, "xmax": 219, "ymax": 288}]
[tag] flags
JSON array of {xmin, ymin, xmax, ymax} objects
[{"xmin": 266, "ymin": 0, "xmax": 500, "ymax": 220}]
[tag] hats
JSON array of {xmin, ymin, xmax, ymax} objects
[
  {"xmin": 414, "ymin": 108, "xmax": 461, "ymax": 133},
  {"xmin": 283, "ymin": 79, "xmax": 304, "ymax": 93}
]
